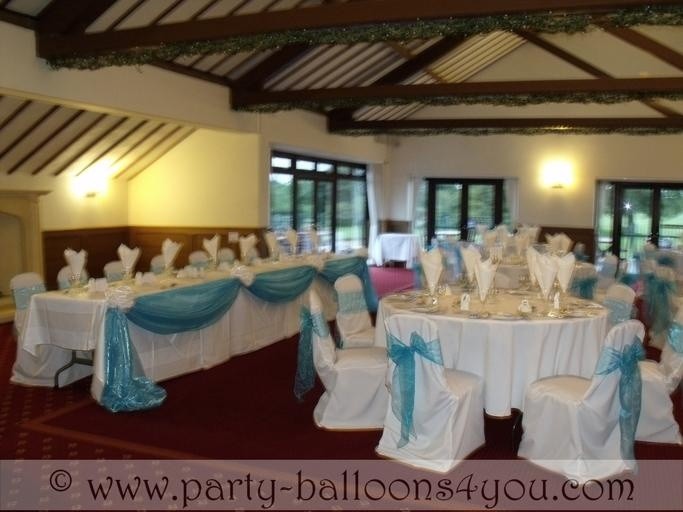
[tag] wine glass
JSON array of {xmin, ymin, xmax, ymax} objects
[
  {"xmin": 540, "ymin": 289, "xmax": 553, "ymax": 313},
  {"xmin": 478, "ymin": 288, "xmax": 489, "ymax": 311},
  {"xmin": 71, "ymin": 273, "xmax": 81, "ymax": 289},
  {"xmin": 122, "ymin": 266, "xmax": 133, "ymax": 280},
  {"xmin": 427, "ymin": 283, "xmax": 438, "ymax": 304}
]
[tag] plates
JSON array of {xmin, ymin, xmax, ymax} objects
[{"xmin": 412, "ymin": 307, "xmax": 429, "ymax": 312}]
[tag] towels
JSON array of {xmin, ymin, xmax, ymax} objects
[
  {"xmin": 161, "ymin": 229, "xmax": 320, "ymax": 270},
  {"xmin": 477, "ymin": 224, "xmax": 572, "ymax": 256}
]
